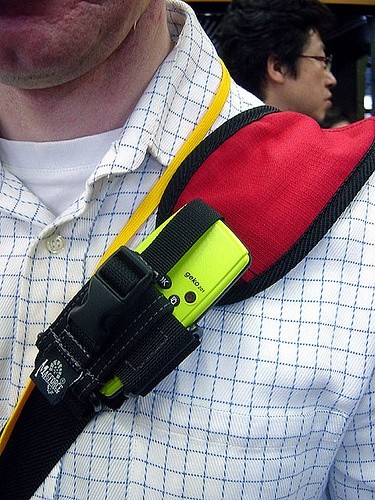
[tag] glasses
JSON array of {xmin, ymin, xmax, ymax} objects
[{"xmin": 293, "ymin": 52, "xmax": 333, "ymax": 70}]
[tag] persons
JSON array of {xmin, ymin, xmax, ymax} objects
[
  {"xmin": 214, "ymin": 7, "xmax": 336, "ymax": 133},
  {"xmin": 0, "ymin": 0, "xmax": 374, "ymax": 500}
]
